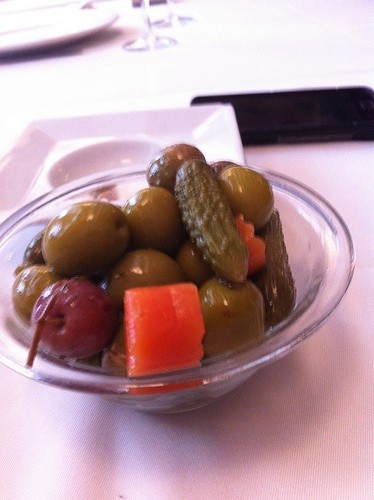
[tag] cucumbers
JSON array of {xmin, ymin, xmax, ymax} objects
[
  {"xmin": 260, "ymin": 209, "xmax": 295, "ymax": 326},
  {"xmin": 176, "ymin": 159, "xmax": 249, "ymax": 283}
]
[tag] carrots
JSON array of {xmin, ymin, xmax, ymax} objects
[
  {"xmin": 122, "ymin": 282, "xmax": 204, "ymax": 377},
  {"xmin": 236, "ymin": 214, "xmax": 266, "ymax": 276}
]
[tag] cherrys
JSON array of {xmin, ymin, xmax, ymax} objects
[{"xmin": 24, "ymin": 277, "xmax": 117, "ymax": 367}]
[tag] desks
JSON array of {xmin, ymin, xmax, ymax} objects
[{"xmin": 0, "ymin": 0, "xmax": 374, "ymax": 500}]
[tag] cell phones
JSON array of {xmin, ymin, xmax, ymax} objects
[{"xmin": 191, "ymin": 86, "xmax": 374, "ymax": 147}]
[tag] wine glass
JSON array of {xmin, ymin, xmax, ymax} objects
[
  {"xmin": 122, "ymin": 0, "xmax": 176, "ymax": 51},
  {"xmin": 152, "ymin": 0, "xmax": 196, "ymax": 29}
]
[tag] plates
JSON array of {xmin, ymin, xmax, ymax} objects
[
  {"xmin": 0, "ymin": 103, "xmax": 247, "ymax": 222},
  {"xmin": 0, "ymin": 7, "xmax": 119, "ymax": 57}
]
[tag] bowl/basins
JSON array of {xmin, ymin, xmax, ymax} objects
[
  {"xmin": 0, "ymin": 160, "xmax": 355, "ymax": 414},
  {"xmin": 48, "ymin": 141, "xmax": 162, "ymax": 191}
]
